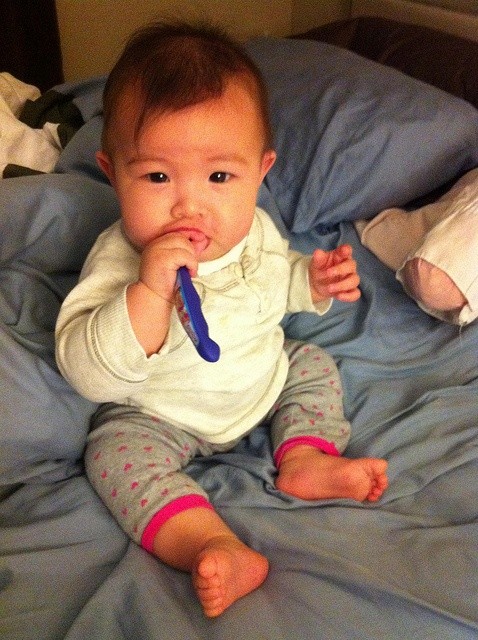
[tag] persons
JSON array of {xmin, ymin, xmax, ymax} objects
[{"xmin": 52, "ymin": 4, "xmax": 390, "ymax": 619}]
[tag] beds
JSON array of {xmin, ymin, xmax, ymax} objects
[{"xmin": 1, "ymin": 32, "xmax": 477, "ymax": 617}]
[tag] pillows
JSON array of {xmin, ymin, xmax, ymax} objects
[{"xmin": 236, "ymin": 33, "xmax": 476, "ymax": 235}]
